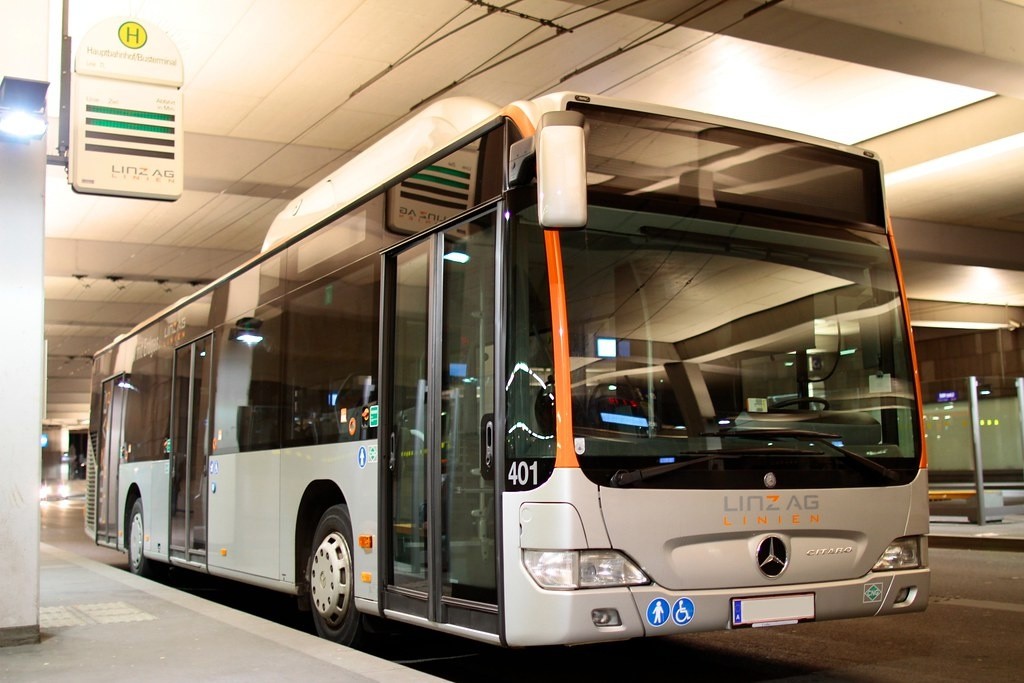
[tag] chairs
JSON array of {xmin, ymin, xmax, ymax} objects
[{"xmin": 251, "ymin": 367, "xmax": 648, "ymax": 442}]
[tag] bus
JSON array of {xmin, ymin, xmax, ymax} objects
[{"xmin": 79, "ymin": 89, "xmax": 934, "ymax": 657}]
